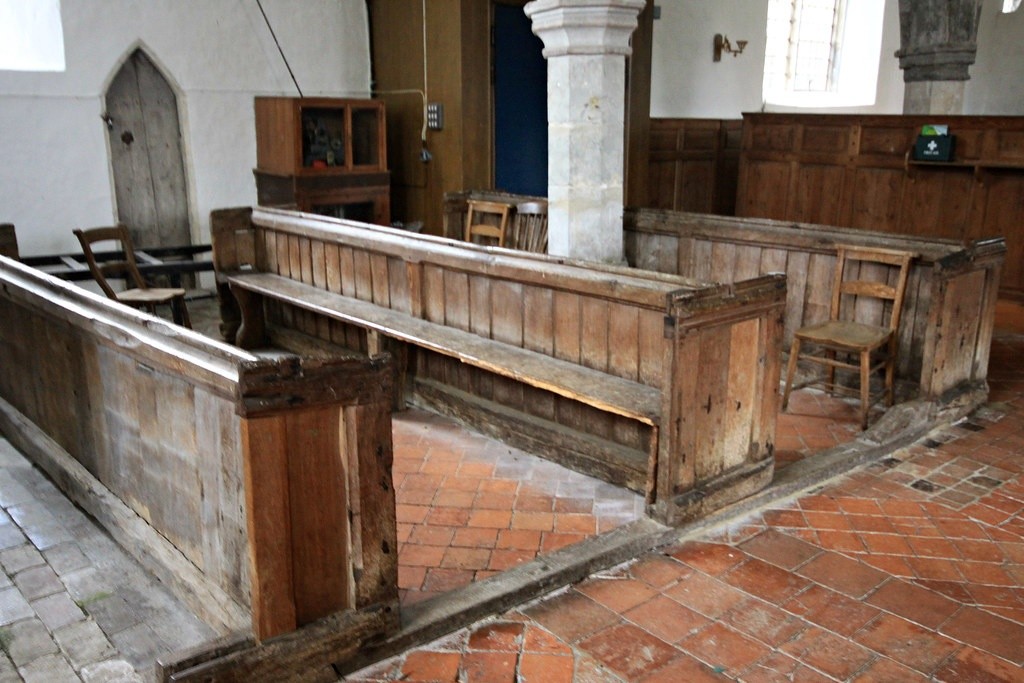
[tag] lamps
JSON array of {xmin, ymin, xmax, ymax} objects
[{"xmin": 714, "ymin": 34, "xmax": 748, "ymax": 62}]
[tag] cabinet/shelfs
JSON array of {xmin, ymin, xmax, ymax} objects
[{"xmin": 253, "ymin": 96, "xmax": 390, "ymax": 227}]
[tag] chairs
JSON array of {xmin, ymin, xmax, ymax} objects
[
  {"xmin": 464, "ymin": 198, "xmax": 548, "ymax": 253},
  {"xmin": 781, "ymin": 242, "xmax": 921, "ymax": 430},
  {"xmin": 72, "ymin": 224, "xmax": 193, "ymax": 331}
]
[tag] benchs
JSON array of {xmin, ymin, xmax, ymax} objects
[
  {"xmin": 0, "ymin": 221, "xmax": 401, "ymax": 643},
  {"xmin": 210, "ymin": 206, "xmax": 788, "ymax": 527},
  {"xmin": 469, "ymin": 188, "xmax": 1008, "ymax": 416}
]
